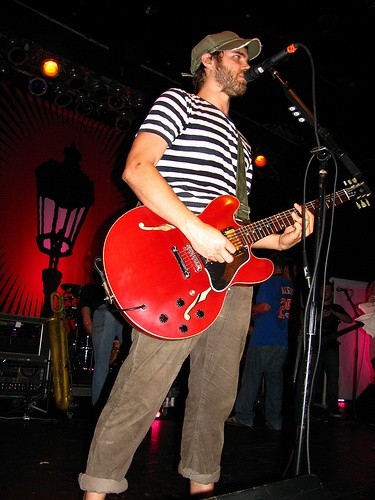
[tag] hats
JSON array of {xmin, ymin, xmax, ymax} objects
[{"xmin": 191, "ymin": 30, "xmax": 262, "ymax": 76}]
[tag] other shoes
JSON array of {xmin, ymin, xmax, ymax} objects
[
  {"xmin": 328, "ymin": 406, "xmax": 342, "ymax": 417},
  {"xmin": 311, "ymin": 405, "xmax": 321, "ymax": 420},
  {"xmin": 225, "ymin": 416, "xmax": 243, "ymax": 426}
]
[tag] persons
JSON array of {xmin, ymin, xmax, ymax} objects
[
  {"xmin": 222, "ymin": 253, "xmax": 295, "ymax": 430},
  {"xmin": 77, "ymin": 31, "xmax": 316, "ymax": 500},
  {"xmin": 80, "ymin": 282, "xmax": 147, "ymax": 409},
  {"xmin": 299, "ymin": 278, "xmax": 352, "ymax": 420}
]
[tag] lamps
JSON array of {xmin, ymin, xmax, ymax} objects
[
  {"xmin": 0, "ymin": 34, "xmax": 141, "ymax": 138},
  {"xmin": 249, "ymin": 141, "xmax": 270, "ymax": 170}
]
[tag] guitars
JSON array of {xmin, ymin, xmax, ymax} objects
[
  {"xmin": 101, "ymin": 175, "xmax": 375, "ymax": 341},
  {"xmin": 315, "ymin": 321, "xmax": 364, "ymax": 356}
]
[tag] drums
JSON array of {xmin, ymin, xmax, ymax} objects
[
  {"xmin": 64, "ymin": 305, "xmax": 82, "ymax": 316},
  {"xmin": 108, "ymin": 339, "xmax": 125, "ymax": 368}
]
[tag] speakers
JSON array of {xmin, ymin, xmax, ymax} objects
[{"xmin": 205, "ymin": 473, "xmax": 338, "ymax": 500}]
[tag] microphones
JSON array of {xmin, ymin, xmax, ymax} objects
[
  {"xmin": 336, "ymin": 287, "xmax": 352, "ymax": 292},
  {"xmin": 244, "ymin": 42, "xmax": 300, "ymax": 84}
]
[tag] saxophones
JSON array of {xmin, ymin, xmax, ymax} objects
[{"xmin": 47, "ymin": 290, "xmax": 72, "ymax": 413}]
